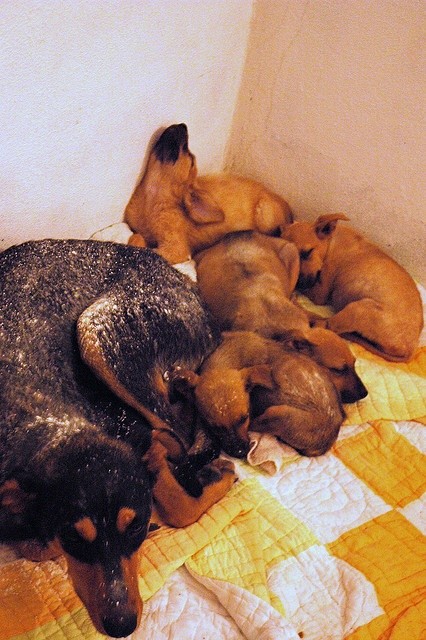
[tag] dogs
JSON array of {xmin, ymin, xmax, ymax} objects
[
  {"xmin": 278, "ymin": 213, "xmax": 424, "ymax": 362},
  {"xmin": 194, "ymin": 229, "xmax": 368, "ymax": 403},
  {"xmin": 125, "ymin": 123, "xmax": 294, "ymax": 267},
  {"xmin": 173, "ymin": 329, "xmax": 345, "ymax": 457},
  {"xmin": 0, "ymin": 238, "xmax": 239, "ymax": 637}
]
